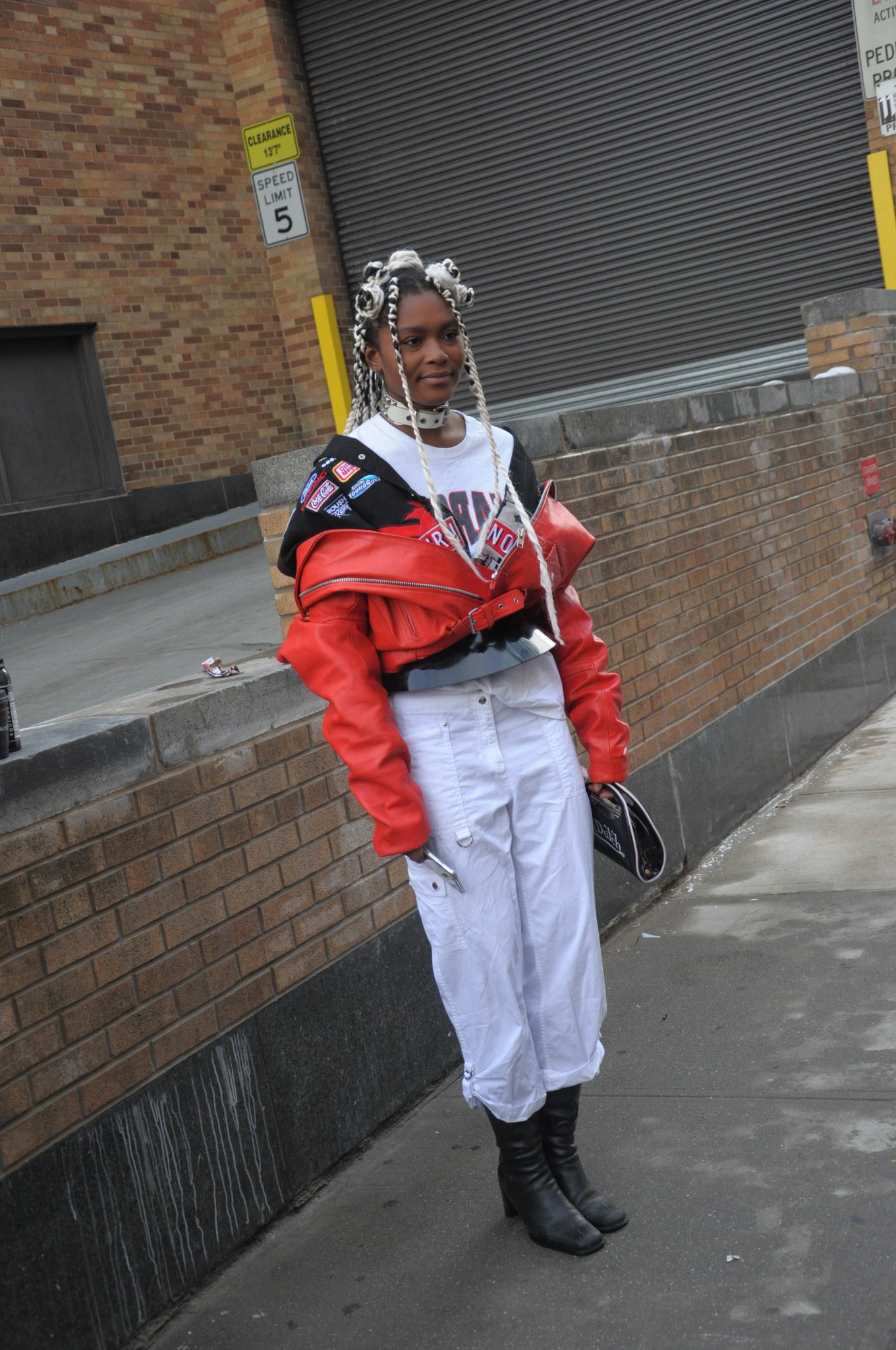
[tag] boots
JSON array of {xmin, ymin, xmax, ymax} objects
[
  {"xmin": 533, "ymin": 1081, "xmax": 629, "ymax": 1233},
  {"xmin": 482, "ymin": 1104, "xmax": 608, "ymax": 1256}
]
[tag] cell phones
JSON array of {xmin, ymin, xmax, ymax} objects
[{"xmin": 423, "ymin": 846, "xmax": 466, "ymax": 894}]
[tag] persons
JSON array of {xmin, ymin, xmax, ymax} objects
[{"xmin": 276, "ymin": 250, "xmax": 631, "ymax": 1256}]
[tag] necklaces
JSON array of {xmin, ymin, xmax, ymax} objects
[{"xmin": 379, "ymin": 383, "xmax": 450, "ymax": 429}]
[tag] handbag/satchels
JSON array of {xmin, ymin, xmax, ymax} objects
[{"xmin": 581, "ymin": 769, "xmax": 664, "ymax": 884}]
